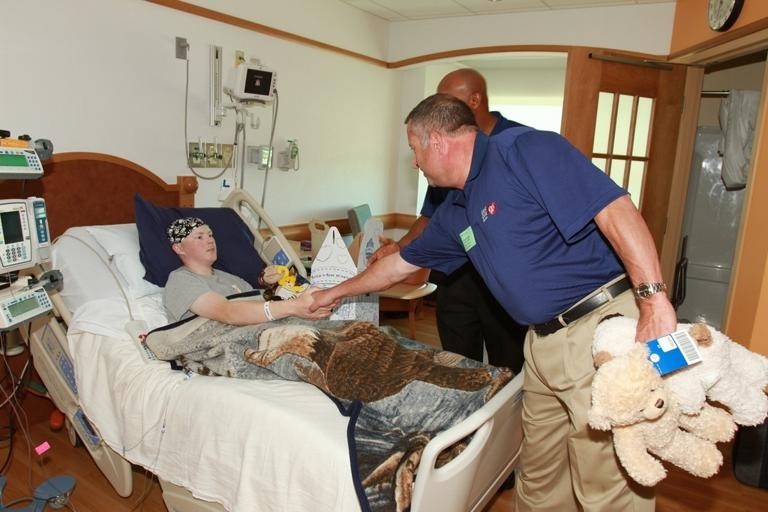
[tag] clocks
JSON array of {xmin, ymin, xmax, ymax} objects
[{"xmin": 706, "ymin": 0, "xmax": 745, "ymax": 33}]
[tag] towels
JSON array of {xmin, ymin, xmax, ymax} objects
[{"xmin": 716, "ymin": 88, "xmax": 766, "ymax": 190}]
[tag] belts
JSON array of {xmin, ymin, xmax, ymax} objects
[{"xmin": 532, "ymin": 275, "xmax": 634, "ymax": 337}]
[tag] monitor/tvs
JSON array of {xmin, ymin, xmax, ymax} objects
[{"xmin": 234, "ymin": 64, "xmax": 277, "ymax": 103}]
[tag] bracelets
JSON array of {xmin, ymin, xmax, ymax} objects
[{"xmin": 261, "ymin": 298, "xmax": 280, "ymax": 323}]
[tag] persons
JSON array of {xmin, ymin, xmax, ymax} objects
[
  {"xmin": 161, "ymin": 215, "xmax": 515, "ymax": 445},
  {"xmin": 307, "ymin": 92, "xmax": 677, "ymax": 512},
  {"xmin": 365, "ymin": 68, "xmax": 526, "ymax": 493}
]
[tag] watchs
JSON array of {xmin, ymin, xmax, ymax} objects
[{"xmin": 631, "ymin": 281, "xmax": 670, "ymax": 301}]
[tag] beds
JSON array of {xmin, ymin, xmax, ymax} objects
[{"xmin": 0, "ymin": 151, "xmax": 530, "ymax": 511}]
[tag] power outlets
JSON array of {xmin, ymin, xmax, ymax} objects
[{"xmin": 174, "ymin": 35, "xmax": 188, "ymax": 61}]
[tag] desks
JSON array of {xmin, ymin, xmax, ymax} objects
[{"xmin": 371, "ymin": 278, "xmax": 438, "ymax": 342}]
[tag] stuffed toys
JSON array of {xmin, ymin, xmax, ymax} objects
[
  {"xmin": 585, "ymin": 342, "xmax": 739, "ymax": 489},
  {"xmin": 591, "ymin": 311, "xmax": 768, "ymax": 427}
]
